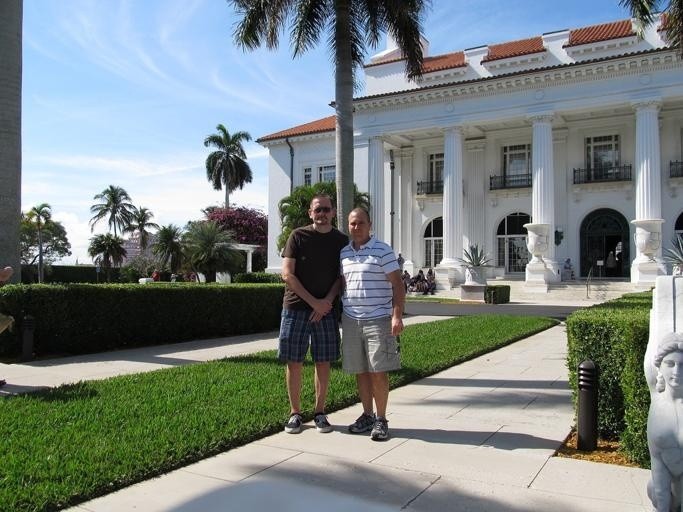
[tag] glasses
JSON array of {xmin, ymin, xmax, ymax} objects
[{"xmin": 309, "ymin": 206, "xmax": 333, "ymax": 213}]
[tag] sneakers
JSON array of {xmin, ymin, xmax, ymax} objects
[
  {"xmin": 348, "ymin": 413, "xmax": 376, "ymax": 433},
  {"xmin": 313, "ymin": 413, "xmax": 331, "ymax": 432},
  {"xmin": 370, "ymin": 416, "xmax": 389, "ymax": 440},
  {"xmin": 284, "ymin": 413, "xmax": 303, "ymax": 433}
]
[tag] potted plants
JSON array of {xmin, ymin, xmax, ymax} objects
[
  {"xmin": 660, "ymin": 235, "xmax": 683, "ymax": 276},
  {"xmin": 457, "ymin": 242, "xmax": 493, "ymax": 287}
]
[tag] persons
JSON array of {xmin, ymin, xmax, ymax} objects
[
  {"xmin": 398, "ymin": 253, "xmax": 436, "ymax": 295},
  {"xmin": 606, "ymin": 251, "xmax": 616, "ymax": 276},
  {"xmin": 647, "ymin": 333, "xmax": 683, "ymax": 512},
  {"xmin": 170, "ymin": 272, "xmax": 177, "ymax": 282},
  {"xmin": 276, "ymin": 192, "xmax": 405, "ymax": 440},
  {"xmin": 564, "ymin": 257, "xmax": 576, "ymax": 280},
  {"xmin": 150, "ymin": 269, "xmax": 160, "ymax": 281}
]
